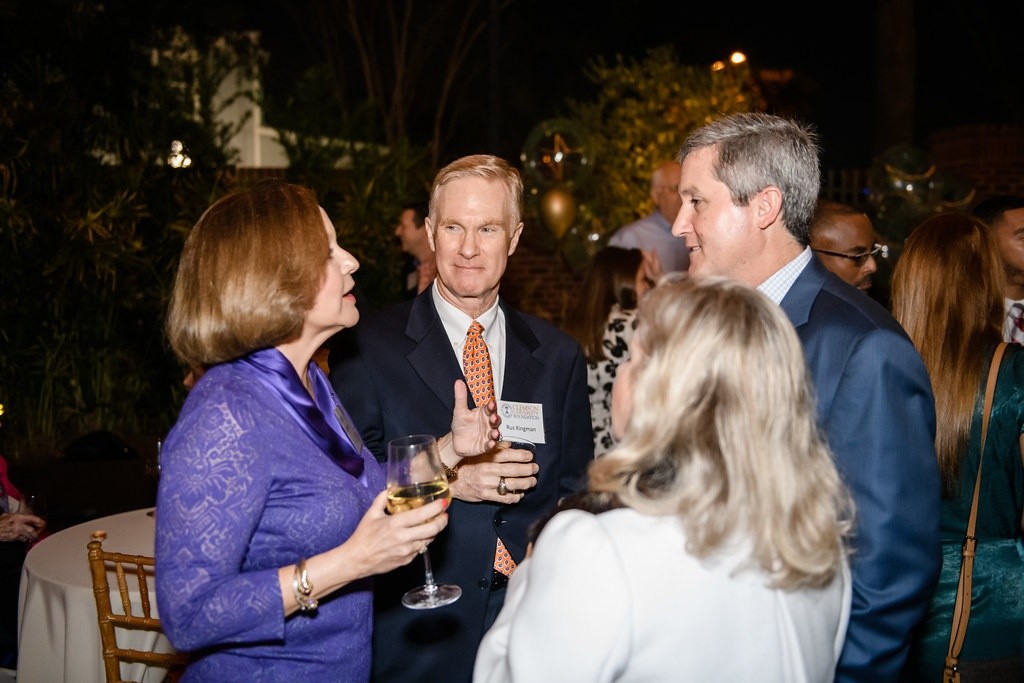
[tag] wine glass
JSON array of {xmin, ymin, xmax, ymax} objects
[
  {"xmin": 144, "ymin": 437, "xmax": 165, "ymax": 518},
  {"xmin": 386, "ymin": 434, "xmax": 463, "ymax": 610}
]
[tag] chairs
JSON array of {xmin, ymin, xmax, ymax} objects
[{"xmin": 86, "ymin": 539, "xmax": 180, "ymax": 683}]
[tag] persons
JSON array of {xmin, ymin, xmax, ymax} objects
[
  {"xmin": 0, "ymin": 456, "xmax": 50, "ymax": 668},
  {"xmin": 609, "ymin": 162, "xmax": 691, "ymax": 274},
  {"xmin": 808, "ymin": 195, "xmax": 1024, "ymax": 683},
  {"xmin": 672, "ymin": 111, "xmax": 942, "ymax": 683},
  {"xmin": 344, "ymin": 202, "xmax": 436, "ymax": 307},
  {"xmin": 155, "ymin": 184, "xmax": 501, "ymax": 683},
  {"xmin": 585, "ymin": 245, "xmax": 665, "ymax": 459},
  {"xmin": 475, "ymin": 280, "xmax": 853, "ymax": 683},
  {"xmin": 326, "ymin": 155, "xmax": 595, "ymax": 683}
]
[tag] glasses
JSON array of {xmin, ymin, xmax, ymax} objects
[{"xmin": 805, "ymin": 243, "xmax": 884, "ymax": 268}]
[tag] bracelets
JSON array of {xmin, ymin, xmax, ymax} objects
[
  {"xmin": 292, "ymin": 558, "xmax": 319, "ymax": 610},
  {"xmin": 426, "ymin": 444, "xmax": 458, "ymax": 481}
]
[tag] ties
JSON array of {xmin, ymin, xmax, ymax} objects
[
  {"xmin": 462, "ymin": 320, "xmax": 517, "ymax": 583},
  {"xmin": 1007, "ymin": 303, "xmax": 1024, "ymax": 346}
]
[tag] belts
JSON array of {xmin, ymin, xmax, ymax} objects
[{"xmin": 487, "ymin": 570, "xmax": 510, "ymax": 591}]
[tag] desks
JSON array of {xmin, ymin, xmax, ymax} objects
[{"xmin": 18, "ymin": 505, "xmax": 205, "ymax": 683}]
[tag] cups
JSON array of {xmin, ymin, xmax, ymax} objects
[
  {"xmin": 490, "ymin": 436, "xmax": 537, "ymax": 496},
  {"xmin": 13, "ymin": 488, "xmax": 48, "ymax": 547}
]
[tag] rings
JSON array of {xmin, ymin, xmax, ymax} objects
[
  {"xmin": 418, "ymin": 539, "xmax": 427, "ymax": 554},
  {"xmin": 498, "ymin": 478, "xmax": 508, "ymax": 495}
]
